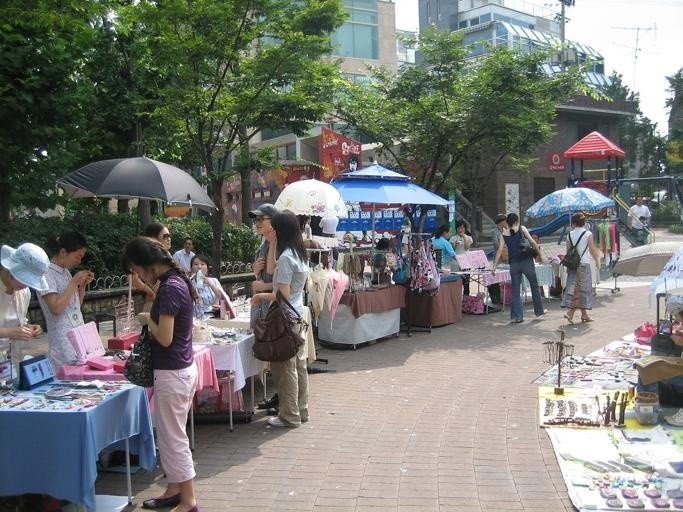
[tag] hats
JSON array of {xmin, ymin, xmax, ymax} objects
[
  {"xmin": 665, "ymin": 295, "xmax": 683, "ymax": 317},
  {"xmin": 0, "ymin": 241, "xmax": 50, "ymax": 291},
  {"xmin": 247, "ymin": 203, "xmax": 277, "ymax": 218}
]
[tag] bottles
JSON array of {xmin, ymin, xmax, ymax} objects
[
  {"xmin": 196, "ymin": 270, "xmax": 203, "ymax": 289},
  {"xmin": 232, "ymin": 294, "xmax": 249, "ymax": 315},
  {"xmin": 219, "ymin": 300, "xmax": 226, "ymax": 320}
]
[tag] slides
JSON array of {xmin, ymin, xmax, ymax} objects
[{"xmin": 486, "ymin": 212, "xmax": 569, "ymax": 258}]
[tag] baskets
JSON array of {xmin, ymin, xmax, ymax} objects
[{"xmin": 637, "ymin": 391, "xmax": 657, "ymax": 402}]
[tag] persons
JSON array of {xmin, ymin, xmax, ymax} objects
[
  {"xmin": 130, "ymin": 222, "xmax": 190, "ymax": 312},
  {"xmin": 171, "ymin": 237, "xmax": 195, "ymax": 273},
  {"xmin": 558, "ymin": 213, "xmax": 600, "ymax": 326},
  {"xmin": 249, "ymin": 210, "xmax": 316, "ymax": 428},
  {"xmin": 491, "ymin": 213, "xmax": 544, "ymax": 324},
  {"xmin": 625, "ymin": 196, "xmax": 651, "ymax": 247},
  {"xmin": 666, "ymin": 295, "xmax": 683, "ymax": 340},
  {"xmin": 303, "ymin": 214, "xmax": 474, "ymax": 298},
  {"xmin": 491, "ymin": 212, "xmax": 510, "ymax": 266},
  {"xmin": 244, "ymin": 202, "xmax": 281, "ymax": 416},
  {"xmin": 186, "ymin": 255, "xmax": 223, "ymax": 319},
  {"xmin": 348, "ymin": 155, "xmax": 359, "ymax": 171},
  {"xmin": 118, "ymin": 235, "xmax": 200, "ymax": 511},
  {"xmin": 0, "ymin": 241, "xmax": 49, "ymax": 395},
  {"xmin": 34, "ymin": 231, "xmax": 94, "ymax": 368},
  {"xmin": 565, "ymin": 172, "xmax": 579, "ymax": 189}
]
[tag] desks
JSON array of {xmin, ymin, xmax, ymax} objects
[
  {"xmin": 1, "ymin": 299, "xmax": 318, "ymax": 512},
  {"xmin": 320, "ymin": 263, "xmax": 557, "ymax": 352}
]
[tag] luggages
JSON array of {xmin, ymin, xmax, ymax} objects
[{"xmin": 651, "ymin": 293, "xmax": 683, "ymax": 408}]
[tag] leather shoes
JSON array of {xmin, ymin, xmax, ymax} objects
[
  {"xmin": 535, "ymin": 309, "xmax": 547, "ymax": 317},
  {"xmin": 187, "ymin": 500, "xmax": 200, "ymax": 511},
  {"xmin": 142, "ymin": 493, "xmax": 180, "ymax": 509}
]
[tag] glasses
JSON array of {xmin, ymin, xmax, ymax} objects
[
  {"xmin": 252, "ymin": 216, "xmax": 272, "ymax": 224},
  {"xmin": 163, "ymin": 233, "xmax": 171, "ymax": 241}
]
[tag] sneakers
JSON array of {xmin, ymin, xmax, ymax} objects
[
  {"xmin": 663, "ymin": 408, "xmax": 683, "ymax": 426},
  {"xmin": 267, "ymin": 417, "xmax": 288, "ymax": 427},
  {"xmin": 257, "ymin": 393, "xmax": 278, "ymax": 414}
]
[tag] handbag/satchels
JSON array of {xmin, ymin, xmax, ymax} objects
[
  {"xmin": 461, "ymin": 292, "xmax": 484, "ymax": 314},
  {"xmin": 519, "ymin": 225, "xmax": 537, "ymax": 258},
  {"xmin": 392, "ymin": 271, "xmax": 406, "ymax": 282},
  {"xmin": 123, "ymin": 325, "xmax": 154, "ymax": 387}
]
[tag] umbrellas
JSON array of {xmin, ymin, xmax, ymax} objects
[
  {"xmin": 305, "ymin": 247, "xmax": 350, "ymax": 334},
  {"xmin": 272, "ymin": 173, "xmax": 349, "ymax": 236},
  {"xmin": 329, "ymin": 158, "xmax": 451, "ymax": 284},
  {"xmin": 53, "ymin": 153, "xmax": 220, "ymax": 234},
  {"xmin": 523, "ymin": 185, "xmax": 615, "ymax": 233}
]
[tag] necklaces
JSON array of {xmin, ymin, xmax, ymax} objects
[{"xmin": 75, "ymin": 323, "xmax": 104, "ymax": 359}]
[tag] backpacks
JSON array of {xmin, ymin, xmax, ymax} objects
[
  {"xmin": 251, "ymin": 288, "xmax": 309, "ymax": 362},
  {"xmin": 559, "ymin": 230, "xmax": 588, "ymax": 270}
]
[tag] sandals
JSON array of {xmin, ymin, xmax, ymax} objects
[
  {"xmin": 563, "ymin": 314, "xmax": 574, "ymax": 325},
  {"xmin": 580, "ymin": 316, "xmax": 594, "ymax": 323}
]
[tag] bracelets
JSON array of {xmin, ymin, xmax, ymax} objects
[
  {"xmin": 141, "ymin": 285, "xmax": 148, "ymax": 292},
  {"xmin": 493, "ymin": 265, "xmax": 496, "ymax": 269}
]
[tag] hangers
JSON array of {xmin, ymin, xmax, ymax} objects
[{"xmin": 586, "ymin": 219, "xmax": 610, "ymax": 224}]
